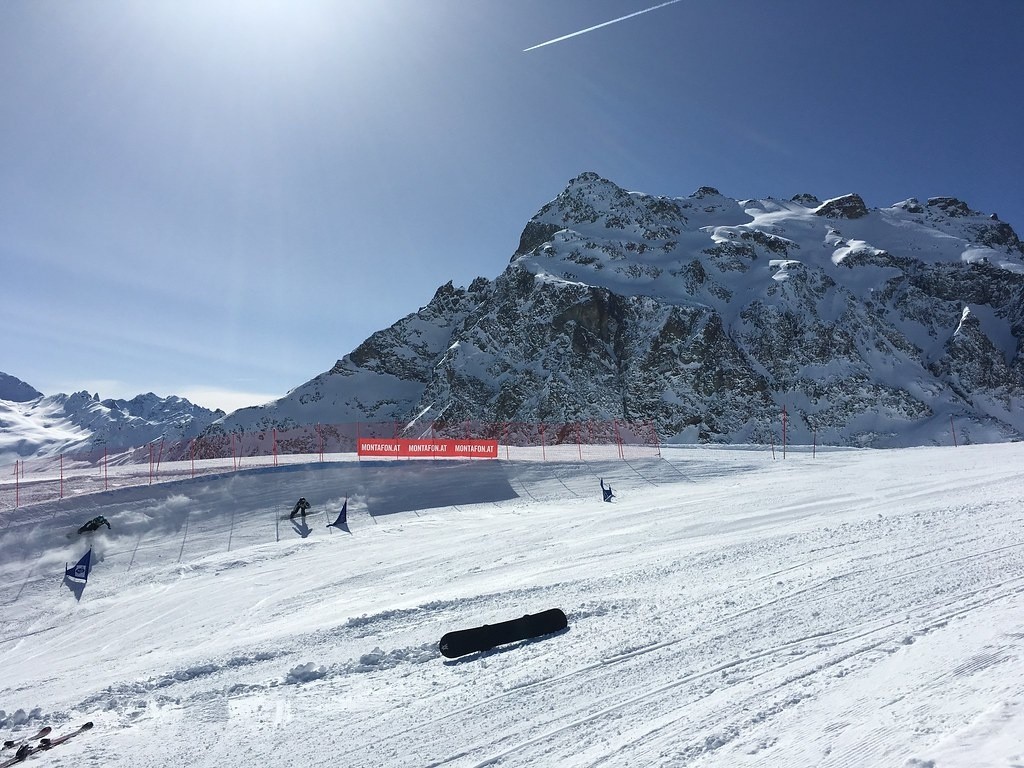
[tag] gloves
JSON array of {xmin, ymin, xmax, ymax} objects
[
  {"xmin": 108, "ymin": 527, "xmax": 111, "ymax": 530},
  {"xmin": 304, "ymin": 506, "xmax": 307, "ymax": 509}
]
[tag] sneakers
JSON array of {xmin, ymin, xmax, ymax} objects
[
  {"xmin": 302, "ymin": 515, "xmax": 306, "ymax": 516},
  {"xmin": 77, "ymin": 530, "xmax": 81, "ymax": 534},
  {"xmin": 290, "ymin": 516, "xmax": 293, "ymax": 519}
]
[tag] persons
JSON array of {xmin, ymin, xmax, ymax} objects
[
  {"xmin": 77, "ymin": 514, "xmax": 111, "ymax": 535},
  {"xmin": 290, "ymin": 497, "xmax": 310, "ymax": 519}
]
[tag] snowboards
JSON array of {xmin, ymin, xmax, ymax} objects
[{"xmin": 438, "ymin": 608, "xmax": 568, "ymax": 660}]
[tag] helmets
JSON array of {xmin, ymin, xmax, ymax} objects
[
  {"xmin": 97, "ymin": 515, "xmax": 104, "ymax": 523},
  {"xmin": 299, "ymin": 497, "xmax": 306, "ymax": 504}
]
